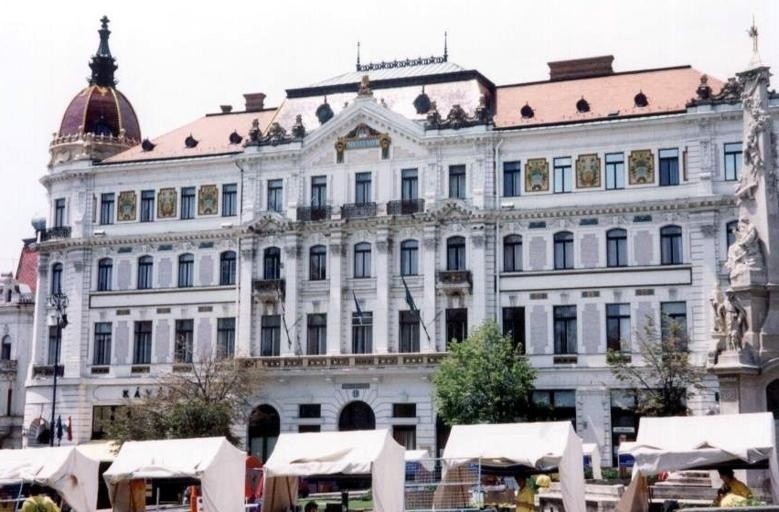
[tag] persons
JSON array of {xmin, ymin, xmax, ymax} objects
[
  {"xmin": 732, "ymin": 172, "xmax": 759, "ymax": 206},
  {"xmin": 742, "ymin": 108, "xmax": 771, "ymax": 170},
  {"xmin": 717, "ymin": 287, "xmax": 748, "ymax": 354},
  {"xmin": 708, "ymin": 461, "xmax": 755, "ymax": 508},
  {"xmin": 304, "ymin": 501, "xmax": 320, "ymax": 511},
  {"xmin": 511, "ymin": 470, "xmax": 535, "ymax": 512},
  {"xmin": 723, "ymin": 216, "xmax": 759, "ymax": 272}
]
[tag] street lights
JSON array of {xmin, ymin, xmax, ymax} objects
[{"xmin": 43, "ymin": 289, "xmax": 72, "ymax": 447}]
